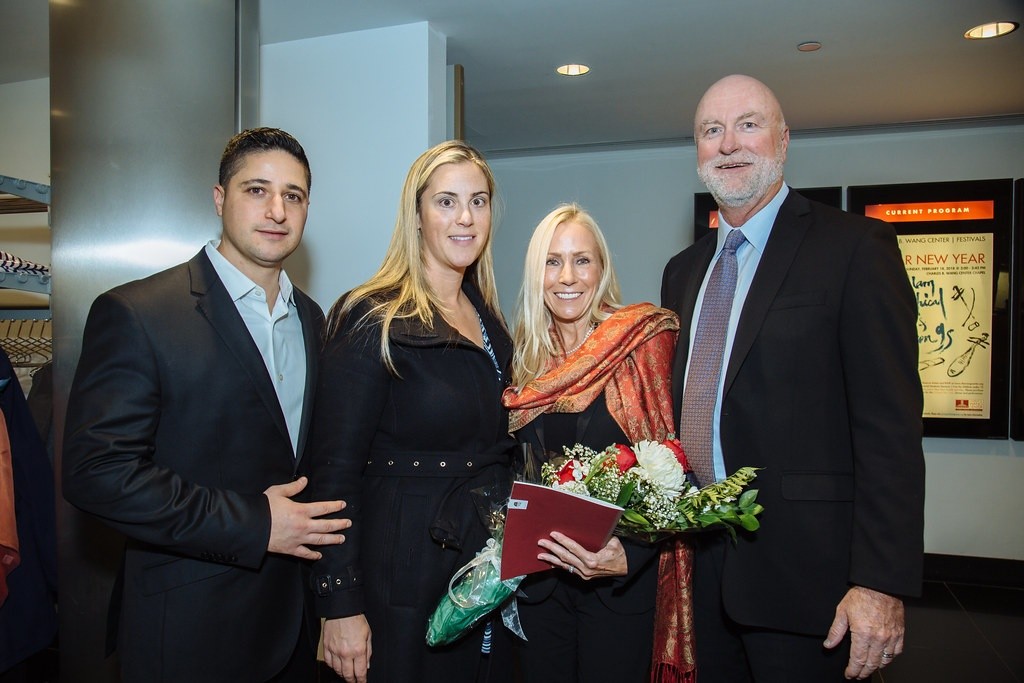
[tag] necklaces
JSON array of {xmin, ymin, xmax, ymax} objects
[{"xmin": 565, "ymin": 321, "xmax": 595, "ymax": 355}]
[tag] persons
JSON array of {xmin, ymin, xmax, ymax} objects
[
  {"xmin": 659, "ymin": 73, "xmax": 927, "ymax": 683},
  {"xmin": 498, "ymin": 201, "xmax": 697, "ymax": 683},
  {"xmin": 310, "ymin": 137, "xmax": 522, "ymax": 683},
  {"xmin": 61, "ymin": 127, "xmax": 353, "ymax": 683}
]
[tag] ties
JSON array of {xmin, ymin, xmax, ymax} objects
[{"xmin": 680, "ymin": 229, "xmax": 745, "ymax": 489}]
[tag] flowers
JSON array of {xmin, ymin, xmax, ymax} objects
[{"xmin": 425, "ymin": 426, "xmax": 766, "ymax": 657}]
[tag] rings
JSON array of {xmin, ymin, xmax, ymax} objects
[
  {"xmin": 882, "ymin": 651, "xmax": 894, "ymax": 659},
  {"xmin": 568, "ymin": 564, "xmax": 575, "ymax": 574}
]
